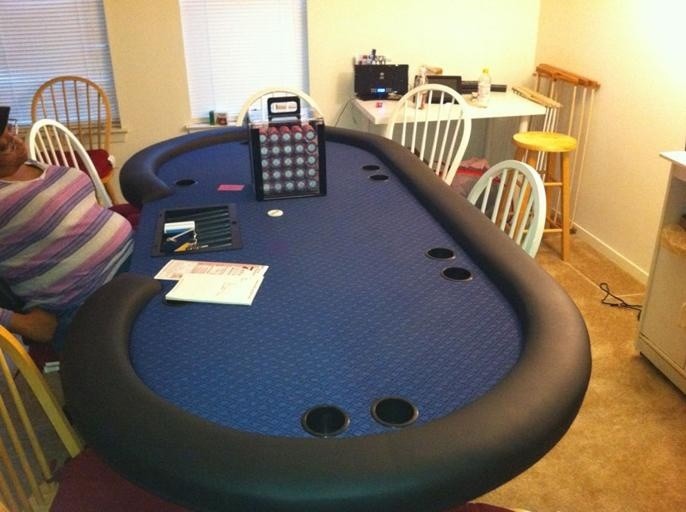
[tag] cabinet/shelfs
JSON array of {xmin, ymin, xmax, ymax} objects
[{"xmin": 633, "ymin": 149, "xmax": 686, "ymax": 398}]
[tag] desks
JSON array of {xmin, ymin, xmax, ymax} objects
[
  {"xmin": 57, "ymin": 123, "xmax": 592, "ymax": 511},
  {"xmin": 347, "ymin": 80, "xmax": 548, "ymax": 165}
]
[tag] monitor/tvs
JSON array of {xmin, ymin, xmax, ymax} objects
[{"xmin": 355, "ymin": 65, "xmax": 408, "ymax": 101}]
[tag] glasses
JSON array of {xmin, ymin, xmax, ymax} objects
[{"xmin": 1, "ymin": 118, "xmax": 19, "ymax": 155}]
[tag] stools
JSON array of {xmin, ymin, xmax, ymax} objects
[{"xmin": 491, "ymin": 131, "xmax": 579, "ymax": 263}]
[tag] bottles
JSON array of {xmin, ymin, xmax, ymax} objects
[
  {"xmin": 414, "ymin": 68, "xmax": 429, "ymax": 110},
  {"xmin": 477, "ymin": 68, "xmax": 491, "ymax": 109}
]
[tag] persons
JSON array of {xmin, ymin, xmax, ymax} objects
[{"xmin": 0, "ymin": 104, "xmax": 138, "ymax": 355}]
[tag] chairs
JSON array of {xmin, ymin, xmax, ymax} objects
[
  {"xmin": 0, "ymin": 325, "xmax": 191, "ymax": 512},
  {"xmin": 23, "ymin": 74, "xmax": 142, "ymax": 232},
  {"xmin": 235, "ymin": 86, "xmax": 328, "ymax": 127},
  {"xmin": 383, "ymin": 82, "xmax": 548, "ymax": 259}
]
